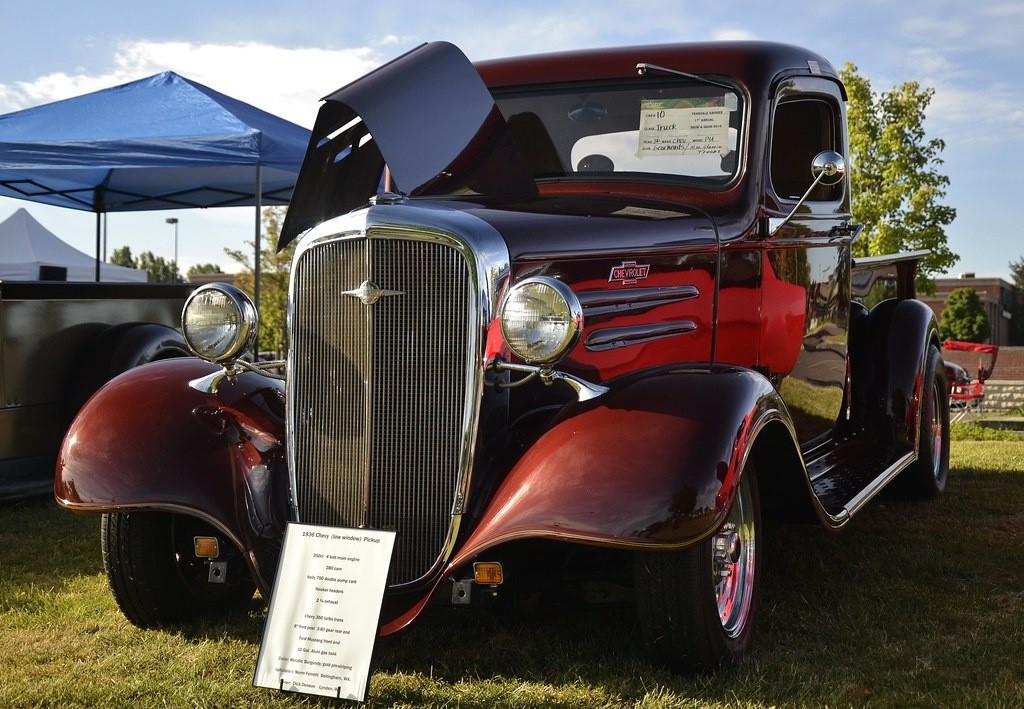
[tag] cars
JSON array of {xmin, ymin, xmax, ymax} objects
[{"xmin": 49, "ymin": 38, "xmax": 1002, "ymax": 677}]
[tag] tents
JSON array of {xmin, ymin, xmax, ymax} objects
[
  {"xmin": 0, "ymin": 70, "xmax": 353, "ymax": 365},
  {"xmin": 0, "ymin": 207, "xmax": 149, "ymax": 283}
]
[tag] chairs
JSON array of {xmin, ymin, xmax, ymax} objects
[{"xmin": 944, "ymin": 340, "xmax": 999, "ymax": 423}]
